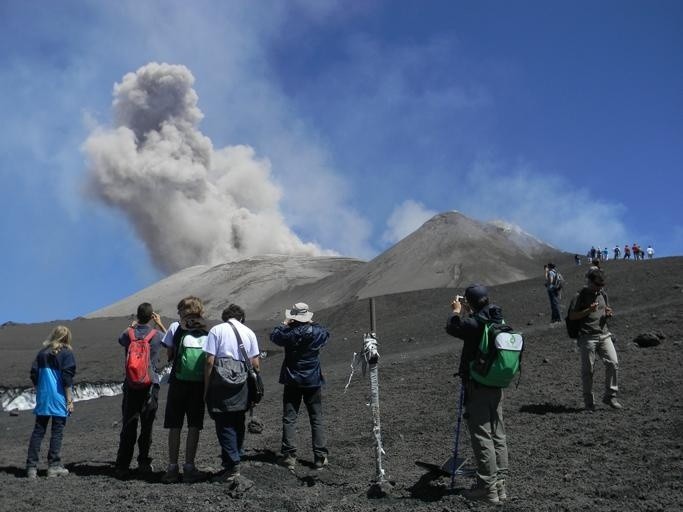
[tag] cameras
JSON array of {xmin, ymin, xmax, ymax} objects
[{"xmin": 458, "ymin": 296, "xmax": 466, "ymax": 303}]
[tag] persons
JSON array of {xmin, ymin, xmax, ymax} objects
[
  {"xmin": 159, "ymin": 297, "xmax": 209, "ymax": 482},
  {"xmin": 270, "ymin": 303, "xmax": 329, "ymax": 470},
  {"xmin": 108, "ymin": 303, "xmax": 168, "ymax": 479},
  {"xmin": 203, "ymin": 305, "xmax": 260, "ymax": 481},
  {"xmin": 575, "ymin": 243, "xmax": 654, "ymax": 270},
  {"xmin": 445, "ymin": 283, "xmax": 509, "ymax": 504},
  {"xmin": 26, "ymin": 325, "xmax": 76, "ymax": 478},
  {"xmin": 544, "ymin": 263, "xmax": 562, "ymax": 323},
  {"xmin": 567, "ymin": 270, "xmax": 622, "ymax": 410}
]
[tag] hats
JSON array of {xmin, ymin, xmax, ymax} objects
[
  {"xmin": 463, "ymin": 283, "xmax": 489, "ymax": 306},
  {"xmin": 285, "ymin": 301, "xmax": 315, "ymax": 323},
  {"xmin": 588, "ymin": 269, "xmax": 607, "ymax": 287}
]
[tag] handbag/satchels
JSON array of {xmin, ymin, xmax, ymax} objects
[{"xmin": 249, "ymin": 367, "xmax": 264, "ymax": 403}]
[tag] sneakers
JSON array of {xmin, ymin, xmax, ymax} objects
[
  {"xmin": 603, "ymin": 391, "xmax": 621, "ymax": 408},
  {"xmin": 112, "ymin": 456, "xmax": 240, "ymax": 486},
  {"xmin": 27, "ymin": 468, "xmax": 37, "ymax": 479},
  {"xmin": 313, "ymin": 451, "xmax": 329, "ymax": 470},
  {"xmin": 277, "ymin": 452, "xmax": 297, "ymax": 470},
  {"xmin": 459, "ymin": 474, "xmax": 500, "ymax": 503},
  {"xmin": 583, "ymin": 393, "xmax": 594, "ymax": 411},
  {"xmin": 496, "ymin": 475, "xmax": 509, "ymax": 501},
  {"xmin": 47, "ymin": 465, "xmax": 70, "ymax": 478}
]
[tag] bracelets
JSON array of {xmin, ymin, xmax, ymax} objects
[
  {"xmin": 67, "ymin": 399, "xmax": 72, "ymax": 405},
  {"xmin": 129, "ymin": 325, "xmax": 135, "ymax": 329}
]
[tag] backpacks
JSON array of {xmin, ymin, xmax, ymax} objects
[
  {"xmin": 122, "ymin": 326, "xmax": 159, "ymax": 391},
  {"xmin": 169, "ymin": 313, "xmax": 209, "ymax": 387},
  {"xmin": 565, "ymin": 288, "xmax": 608, "ymax": 339},
  {"xmin": 466, "ymin": 316, "xmax": 525, "ymax": 389}
]
